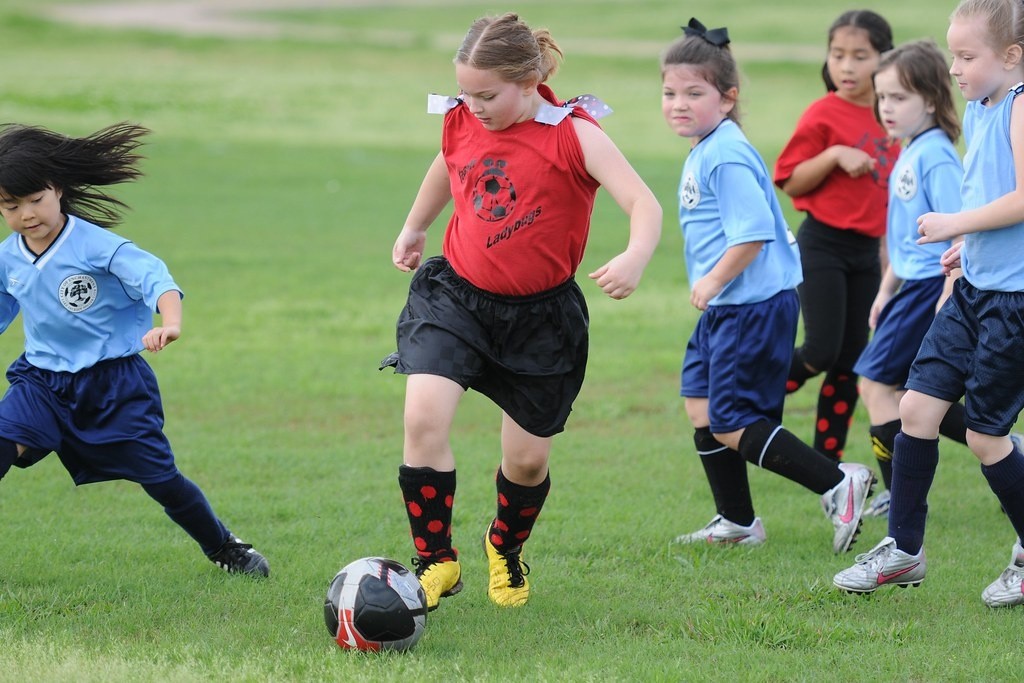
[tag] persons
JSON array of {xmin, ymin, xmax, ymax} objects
[
  {"xmin": 775, "ymin": 10, "xmax": 895, "ymax": 461},
  {"xmin": 661, "ymin": 18, "xmax": 876, "ymax": 553},
  {"xmin": 378, "ymin": 14, "xmax": 664, "ymax": 612},
  {"xmin": 0, "ymin": 120, "xmax": 268, "ymax": 579},
  {"xmin": 832, "ymin": 0, "xmax": 1024, "ymax": 608}
]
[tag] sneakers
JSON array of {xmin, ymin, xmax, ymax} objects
[
  {"xmin": 410, "ymin": 548, "xmax": 464, "ymax": 609},
  {"xmin": 675, "ymin": 515, "xmax": 767, "ymax": 550},
  {"xmin": 208, "ymin": 534, "xmax": 272, "ymax": 579},
  {"xmin": 831, "ymin": 537, "xmax": 926, "ymax": 597},
  {"xmin": 818, "ymin": 460, "xmax": 878, "ymax": 556},
  {"xmin": 863, "ymin": 488, "xmax": 891, "ymax": 519},
  {"xmin": 980, "ymin": 536, "xmax": 1024, "ymax": 608},
  {"xmin": 481, "ymin": 516, "xmax": 530, "ymax": 609}
]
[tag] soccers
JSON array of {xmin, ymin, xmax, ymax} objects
[{"xmin": 326, "ymin": 558, "xmax": 428, "ymax": 656}]
